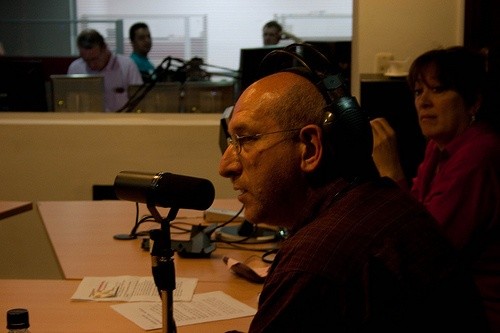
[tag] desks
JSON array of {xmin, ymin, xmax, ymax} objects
[
  {"xmin": 128, "ymin": 79, "xmax": 234, "ymax": 114},
  {"xmin": 0, "ymin": 188, "xmax": 282, "ymax": 333}
]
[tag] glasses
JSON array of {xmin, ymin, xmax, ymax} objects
[{"xmin": 227, "ymin": 128, "xmax": 301, "ymax": 155}]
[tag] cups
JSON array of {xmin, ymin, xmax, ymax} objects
[
  {"xmin": 389, "ymin": 61, "xmax": 407, "ymax": 73},
  {"xmin": 376, "ymin": 53, "xmax": 394, "ymax": 73}
]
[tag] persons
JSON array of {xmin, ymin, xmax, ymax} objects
[
  {"xmin": 369, "ymin": 45, "xmax": 500, "ymax": 244},
  {"xmin": 218, "ymin": 67, "xmax": 491, "ymax": 333},
  {"xmin": 67, "ymin": 31, "xmax": 142, "ymax": 112},
  {"xmin": 130, "ymin": 23, "xmax": 155, "ymax": 74},
  {"xmin": 263, "ymin": 22, "xmax": 302, "ymax": 47}
]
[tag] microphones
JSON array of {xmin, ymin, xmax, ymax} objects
[{"xmin": 114, "ymin": 169, "xmax": 216, "ymax": 211}]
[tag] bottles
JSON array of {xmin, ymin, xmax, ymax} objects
[{"xmin": 7, "ymin": 308, "xmax": 32, "ymax": 333}]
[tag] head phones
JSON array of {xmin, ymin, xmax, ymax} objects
[{"xmin": 260, "ymin": 42, "xmax": 373, "ymax": 176}]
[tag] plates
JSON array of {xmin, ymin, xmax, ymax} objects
[{"xmin": 383, "ymin": 72, "xmax": 409, "ymax": 77}]
